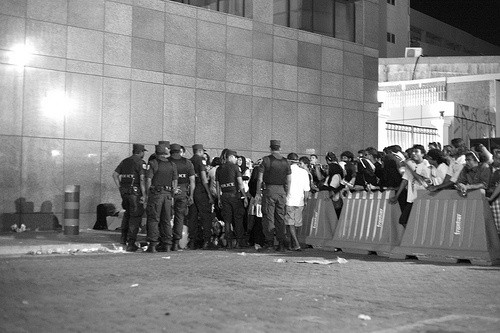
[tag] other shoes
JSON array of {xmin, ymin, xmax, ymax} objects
[
  {"xmin": 115, "ymin": 233, "xmax": 289, "ymax": 255},
  {"xmin": 288, "ymin": 246, "xmax": 302, "ymax": 251}
]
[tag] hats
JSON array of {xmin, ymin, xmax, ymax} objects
[
  {"xmin": 192, "ymin": 144, "xmax": 206, "ymax": 151},
  {"xmin": 270, "ymin": 139, "xmax": 282, "ymax": 148},
  {"xmin": 226, "ymin": 151, "xmax": 239, "ymax": 157},
  {"xmin": 132, "ymin": 144, "xmax": 148, "ymax": 153},
  {"xmin": 155, "ymin": 144, "xmax": 171, "ymax": 155},
  {"xmin": 169, "ymin": 144, "xmax": 182, "ymax": 153},
  {"xmin": 158, "ymin": 141, "xmax": 171, "ymax": 149}
]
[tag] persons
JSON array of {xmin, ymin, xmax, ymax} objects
[{"xmin": 107, "ymin": 137, "xmax": 500, "ymax": 267}]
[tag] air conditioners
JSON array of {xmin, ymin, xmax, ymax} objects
[{"xmin": 404, "ymin": 47, "xmax": 423, "ymax": 58}]
